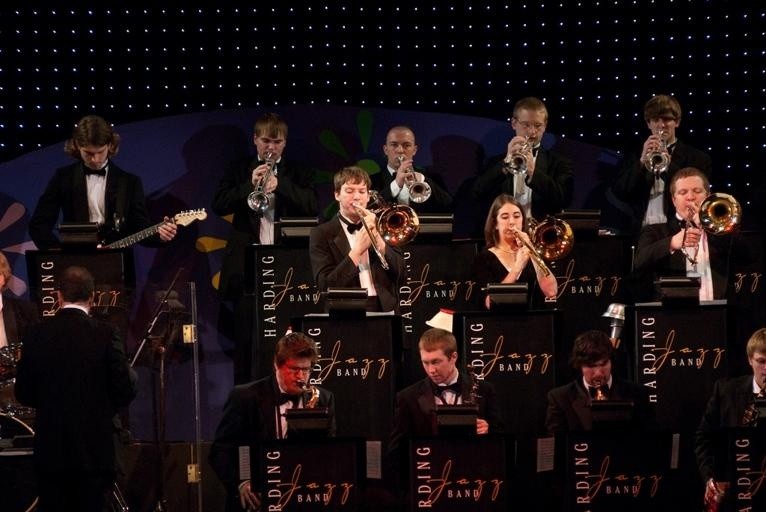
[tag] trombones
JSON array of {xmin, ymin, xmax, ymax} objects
[
  {"xmin": 350, "ymin": 190, "xmax": 419, "ymax": 270},
  {"xmin": 507, "ymin": 216, "xmax": 576, "ymax": 275},
  {"xmin": 681, "ymin": 193, "xmax": 742, "ymax": 265}
]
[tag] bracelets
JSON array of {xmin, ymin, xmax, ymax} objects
[{"xmin": 235, "ymin": 479, "xmax": 251, "ymax": 493}]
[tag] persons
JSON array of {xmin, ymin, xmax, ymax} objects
[
  {"xmin": 629, "ymin": 165, "xmax": 748, "ymax": 304},
  {"xmin": 469, "ymin": 95, "xmax": 574, "ymax": 235},
  {"xmin": 469, "ymin": 193, "xmax": 561, "ymax": 314},
  {"xmin": 23, "ymin": 113, "xmax": 179, "ymax": 322},
  {"xmin": 603, "ymin": 91, "xmax": 707, "ymax": 301},
  {"xmin": 380, "ymin": 328, "xmax": 502, "ymax": 511},
  {"xmin": 367, "ymin": 123, "xmax": 455, "ymax": 216},
  {"xmin": 0, "ymin": 252, "xmax": 39, "ymax": 347},
  {"xmin": 306, "ymin": 163, "xmax": 410, "ymax": 393},
  {"xmin": 209, "ymin": 114, "xmax": 317, "ymax": 384},
  {"xmin": 204, "ymin": 333, "xmax": 340, "ymax": 510},
  {"xmin": 541, "ymin": 328, "xmax": 647, "ymax": 511},
  {"xmin": 692, "ymin": 327, "xmax": 766, "ymax": 512},
  {"xmin": 13, "ymin": 263, "xmax": 139, "ymax": 510}
]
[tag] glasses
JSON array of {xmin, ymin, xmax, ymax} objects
[
  {"xmin": 753, "ymin": 356, "xmax": 766, "ymax": 366},
  {"xmin": 283, "ymin": 365, "xmax": 311, "ymax": 375},
  {"xmin": 513, "ymin": 116, "xmax": 546, "ymax": 129}
]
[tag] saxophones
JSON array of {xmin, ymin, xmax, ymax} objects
[
  {"xmin": 249, "ymin": 381, "xmax": 320, "ymax": 512},
  {"xmin": 708, "ymin": 379, "xmax": 766, "ymax": 512}
]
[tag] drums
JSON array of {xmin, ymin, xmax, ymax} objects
[{"xmin": 0, "ymin": 343, "xmax": 36, "ymax": 421}]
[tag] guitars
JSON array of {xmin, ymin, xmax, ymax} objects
[{"xmin": 100, "ymin": 209, "xmax": 208, "ymax": 250}]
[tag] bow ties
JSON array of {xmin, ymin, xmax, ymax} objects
[
  {"xmin": 273, "ymin": 393, "xmax": 302, "ymax": 405},
  {"xmin": 432, "ymin": 382, "xmax": 462, "ymax": 395},
  {"xmin": 257, "ymin": 160, "xmax": 276, "ymax": 172},
  {"xmin": 530, "ymin": 147, "xmax": 538, "ymax": 156},
  {"xmin": 588, "ymin": 383, "xmax": 610, "ymax": 398},
  {"xmin": 392, "ymin": 172, "xmax": 398, "ymax": 180},
  {"xmin": 666, "ymin": 147, "xmax": 673, "ymax": 154},
  {"xmin": 83, "ymin": 164, "xmax": 106, "ymax": 176},
  {"xmin": 678, "ymin": 219, "xmax": 696, "ymax": 230},
  {"xmin": 347, "ymin": 222, "xmax": 363, "ymax": 233}
]
